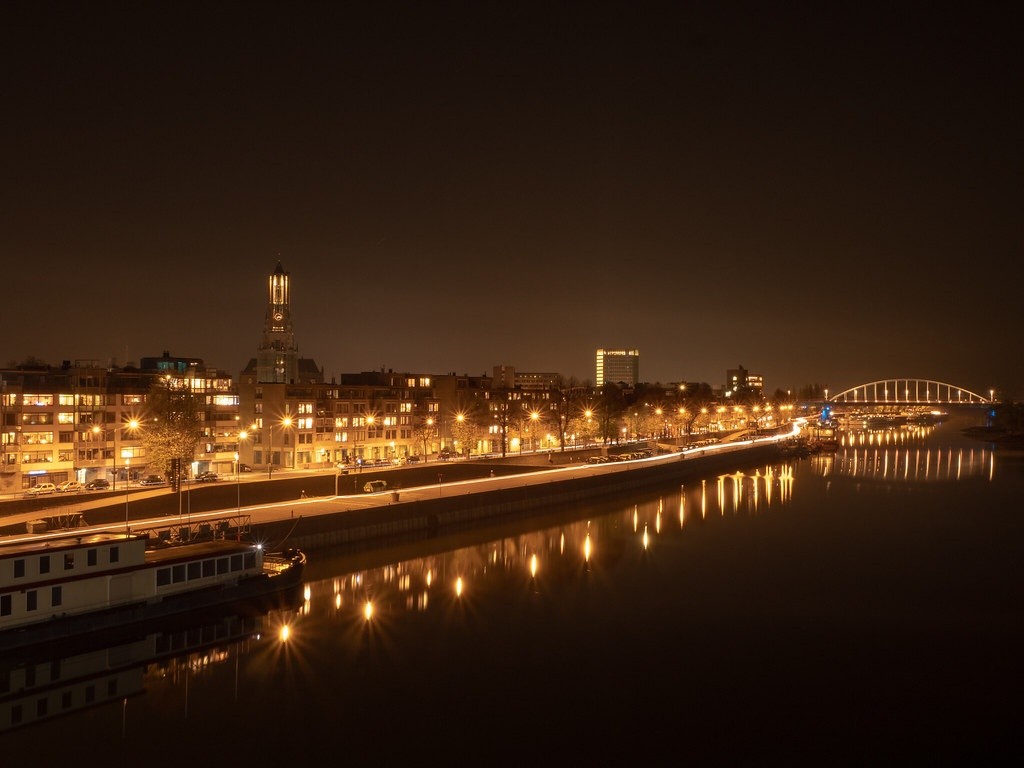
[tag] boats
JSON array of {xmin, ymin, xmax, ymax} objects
[
  {"xmin": 863, "ymin": 411, "xmax": 952, "ymax": 430},
  {"xmin": 1, "ymin": 530, "xmax": 309, "ymax": 651},
  {"xmin": 779, "ymin": 426, "xmax": 845, "ymax": 457}
]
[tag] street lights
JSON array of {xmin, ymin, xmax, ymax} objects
[
  {"xmin": 125, "ymin": 459, "xmax": 130, "ymax": 529},
  {"xmin": 235, "ymin": 453, "xmax": 241, "ymax": 516}
]
